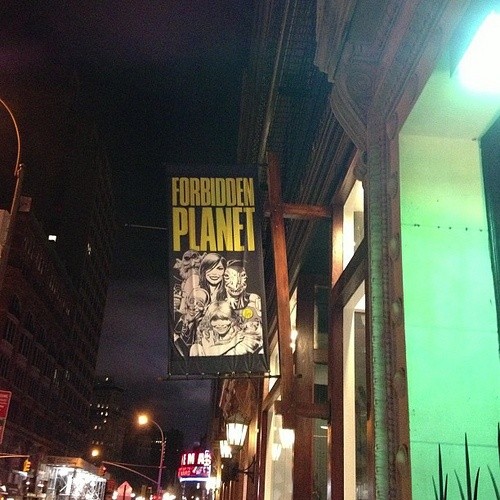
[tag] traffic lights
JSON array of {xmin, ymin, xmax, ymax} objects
[{"xmin": 23, "ymin": 461, "xmax": 31, "ymax": 472}]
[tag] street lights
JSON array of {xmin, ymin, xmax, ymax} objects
[{"xmin": 138, "ymin": 415, "xmax": 167, "ymax": 500}]
